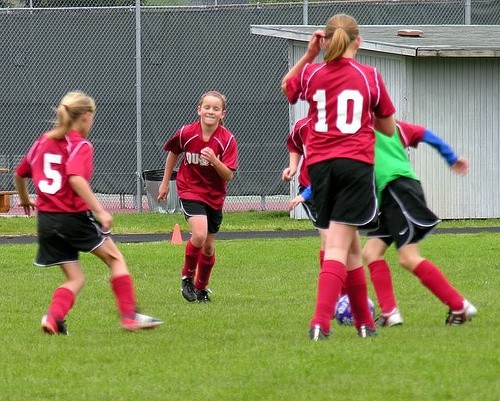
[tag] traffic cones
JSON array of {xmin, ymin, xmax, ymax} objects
[{"xmin": 171, "ymin": 224, "xmax": 182, "ymax": 243}]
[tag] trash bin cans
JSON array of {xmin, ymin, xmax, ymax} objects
[{"xmin": 141, "ymin": 169, "xmax": 182, "ymax": 214}]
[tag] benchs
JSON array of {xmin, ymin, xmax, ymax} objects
[{"xmin": 0, "ymin": 190, "xmax": 19, "ymax": 213}]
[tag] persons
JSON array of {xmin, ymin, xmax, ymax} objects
[
  {"xmin": 157, "ymin": 90, "xmax": 238, "ymax": 303},
  {"xmin": 280, "ymin": 12, "xmax": 479, "ymax": 343},
  {"xmin": 12, "ymin": 89, "xmax": 162, "ymax": 336}
]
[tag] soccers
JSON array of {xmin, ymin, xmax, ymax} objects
[{"xmin": 335, "ymin": 294, "xmax": 376, "ymax": 326}]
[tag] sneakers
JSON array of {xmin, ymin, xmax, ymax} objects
[
  {"xmin": 359, "ymin": 325, "xmax": 377, "ymax": 338},
  {"xmin": 310, "ymin": 326, "xmax": 331, "ymax": 341},
  {"xmin": 375, "ymin": 308, "xmax": 402, "ymax": 328},
  {"xmin": 41, "ymin": 314, "xmax": 70, "ymax": 335},
  {"xmin": 445, "ymin": 299, "xmax": 477, "ymax": 325},
  {"xmin": 119, "ymin": 312, "xmax": 162, "ymax": 330},
  {"xmin": 196, "ymin": 285, "xmax": 212, "ymax": 302},
  {"xmin": 179, "ymin": 275, "xmax": 197, "ymax": 302}
]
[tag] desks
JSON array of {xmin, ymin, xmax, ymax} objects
[{"xmin": 0, "ymin": 168, "xmax": 8, "ymax": 173}]
